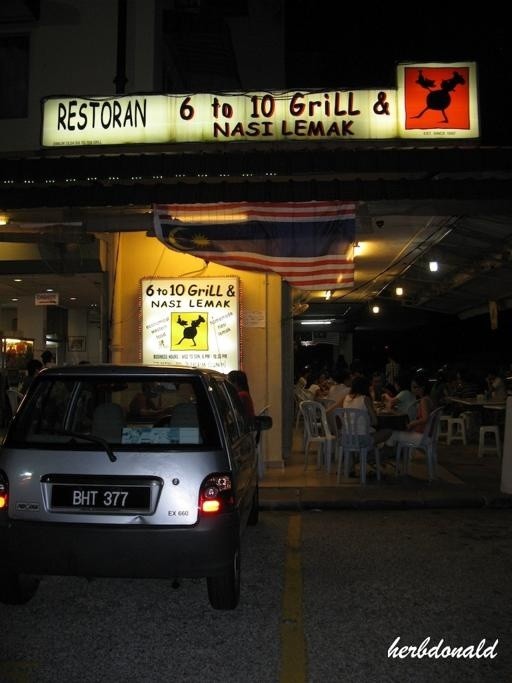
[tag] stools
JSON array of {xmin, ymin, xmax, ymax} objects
[{"xmin": 436, "ymin": 414, "xmax": 502, "ymax": 457}]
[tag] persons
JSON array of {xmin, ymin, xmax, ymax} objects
[
  {"xmin": 128, "ymin": 381, "xmax": 174, "ymax": 416},
  {"xmin": 295, "ymin": 354, "xmax": 512, "ymax": 477},
  {"xmin": 229, "ymin": 372, "xmax": 256, "ymax": 419},
  {"xmin": 18, "ymin": 351, "xmax": 91, "ymax": 410}
]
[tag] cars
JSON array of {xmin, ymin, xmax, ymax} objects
[{"xmin": 0, "ymin": 364, "xmax": 273, "ymax": 614}]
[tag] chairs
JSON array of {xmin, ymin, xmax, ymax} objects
[
  {"xmin": 89, "ymin": 403, "xmax": 125, "ymax": 444},
  {"xmin": 296, "ymin": 382, "xmax": 434, "ymax": 491}
]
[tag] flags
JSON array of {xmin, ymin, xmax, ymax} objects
[{"xmin": 153, "ymin": 201, "xmax": 356, "ymax": 291}]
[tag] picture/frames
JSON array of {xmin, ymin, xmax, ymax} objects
[{"xmin": 138, "ymin": 274, "xmax": 241, "ymax": 377}]
